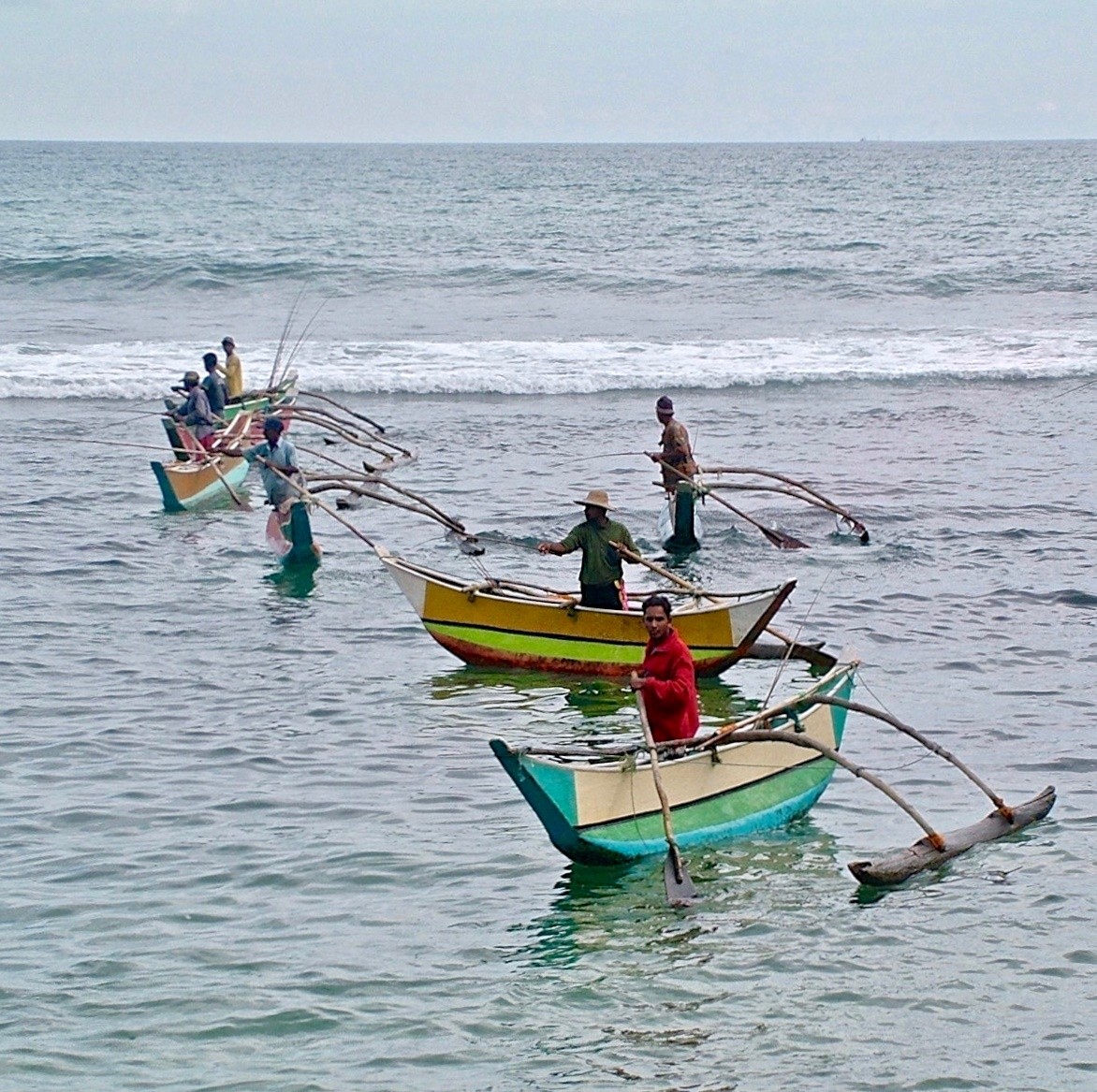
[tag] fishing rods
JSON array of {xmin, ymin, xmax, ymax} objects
[{"xmin": 102, "ymin": 392, "xmax": 185, "ymax": 428}]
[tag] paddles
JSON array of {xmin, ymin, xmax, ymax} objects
[
  {"xmin": 251, "ymin": 446, "xmax": 396, "ymax": 558},
  {"xmin": 629, "ymin": 666, "xmax": 699, "ymax": 910},
  {"xmin": 172, "ymin": 412, "xmax": 251, "ymax": 513},
  {"xmin": 642, "ymin": 447, "xmax": 808, "ymax": 549},
  {"xmin": 608, "ymin": 537, "xmax": 842, "ymax": 671}
]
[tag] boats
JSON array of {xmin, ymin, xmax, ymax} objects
[
  {"xmin": 148, "ymin": 368, "xmax": 299, "ymax": 517},
  {"xmin": 380, "ymin": 553, "xmax": 797, "ymax": 681},
  {"xmin": 488, "ymin": 660, "xmax": 860, "ymax": 869}
]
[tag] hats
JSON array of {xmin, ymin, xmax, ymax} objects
[
  {"xmin": 574, "ymin": 491, "xmax": 615, "ymax": 511},
  {"xmin": 223, "ymin": 337, "xmax": 235, "ymax": 346},
  {"xmin": 657, "ymin": 396, "xmax": 673, "ymax": 413},
  {"xmin": 181, "ymin": 371, "xmax": 199, "ymax": 382},
  {"xmin": 264, "ymin": 418, "xmax": 284, "ymax": 434}
]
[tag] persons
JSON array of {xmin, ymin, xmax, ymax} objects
[
  {"xmin": 537, "ymin": 491, "xmax": 641, "ymax": 610},
  {"xmin": 166, "ymin": 371, "xmax": 216, "ymax": 461},
  {"xmin": 171, "ymin": 353, "xmax": 225, "ymax": 413},
  {"xmin": 628, "ymin": 596, "xmax": 700, "ymax": 742},
  {"xmin": 647, "ymin": 396, "xmax": 697, "ymax": 495},
  {"xmin": 215, "ymin": 337, "xmax": 242, "ymax": 399},
  {"xmin": 214, "ymin": 419, "xmax": 304, "ymax": 505}
]
